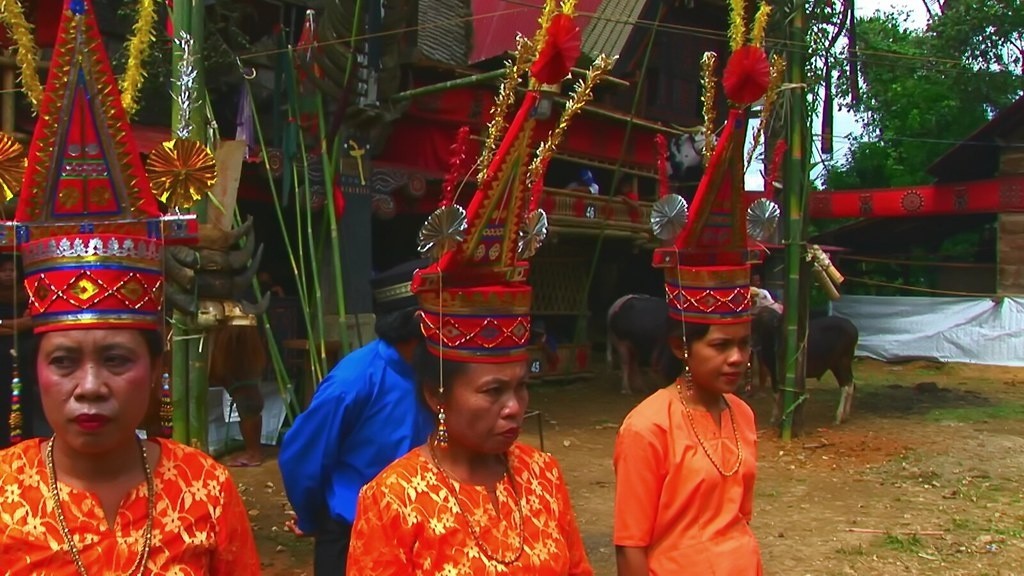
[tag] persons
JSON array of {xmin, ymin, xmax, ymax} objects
[
  {"xmin": 223, "ymin": 383, "xmax": 262, "ymax": 468},
  {"xmin": 611, "ymin": 266, "xmax": 806, "ymax": 576},
  {"xmin": 344, "ymin": 284, "xmax": 623, "ymax": 576},
  {"xmin": 278, "ymin": 260, "xmax": 439, "ymax": 576},
  {"xmin": 0, "ymin": 231, "xmax": 263, "ymax": 576}
]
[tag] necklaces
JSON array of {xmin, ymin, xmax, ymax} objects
[
  {"xmin": 46, "ymin": 432, "xmax": 154, "ymax": 576},
  {"xmin": 427, "ymin": 433, "xmax": 524, "ymax": 563},
  {"xmin": 677, "ymin": 376, "xmax": 742, "ymax": 477}
]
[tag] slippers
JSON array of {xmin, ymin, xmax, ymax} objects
[{"xmin": 228, "ymin": 459, "xmax": 263, "ymax": 467}]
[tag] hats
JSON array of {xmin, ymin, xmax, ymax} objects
[
  {"xmin": 409, "ymin": 0, "xmax": 619, "ymax": 448},
  {"xmin": 649, "ymin": 0, "xmax": 788, "ymax": 396},
  {"xmin": 369, "ymin": 257, "xmax": 440, "ymax": 315},
  {"xmin": 0, "ymin": 0, "xmax": 217, "ymax": 443}
]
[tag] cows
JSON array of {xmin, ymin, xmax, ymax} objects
[
  {"xmin": 606, "ymin": 285, "xmax": 784, "ymax": 396},
  {"xmin": 751, "ymin": 305, "xmax": 859, "ymax": 425}
]
